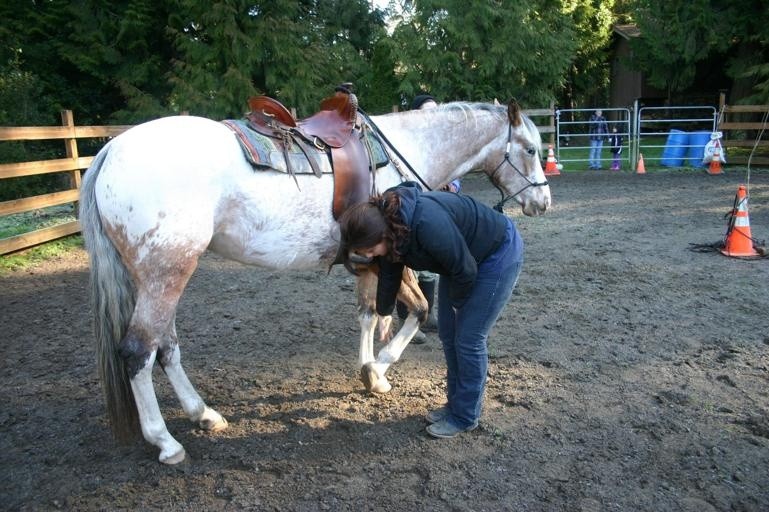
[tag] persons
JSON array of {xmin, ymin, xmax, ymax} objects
[
  {"xmin": 610, "ymin": 127, "xmax": 623, "ymax": 170},
  {"xmin": 588, "ymin": 110, "xmax": 610, "ymax": 170},
  {"xmin": 332, "ymin": 182, "xmax": 523, "ymax": 438},
  {"xmin": 397, "ymin": 95, "xmax": 461, "ymax": 344}
]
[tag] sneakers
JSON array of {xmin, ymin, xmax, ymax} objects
[
  {"xmin": 609, "ymin": 166, "xmax": 619, "ymax": 170},
  {"xmin": 425, "ymin": 407, "xmax": 478, "ymax": 438}
]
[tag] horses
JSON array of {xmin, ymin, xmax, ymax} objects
[{"xmin": 77, "ymin": 96, "xmax": 553, "ymax": 465}]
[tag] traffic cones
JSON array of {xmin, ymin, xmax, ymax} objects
[
  {"xmin": 704, "ymin": 141, "xmax": 726, "ymax": 176},
  {"xmin": 542, "ymin": 144, "xmax": 562, "ymax": 177},
  {"xmin": 635, "ymin": 152, "xmax": 647, "ymax": 174},
  {"xmin": 715, "ymin": 184, "xmax": 762, "ymax": 259}
]
